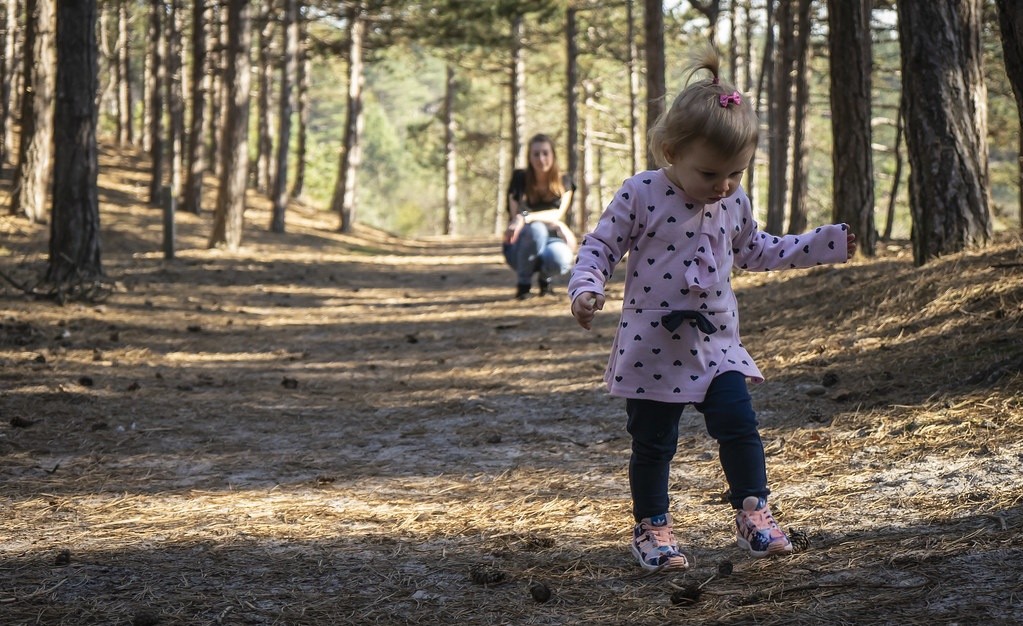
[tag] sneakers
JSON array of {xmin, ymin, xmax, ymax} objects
[
  {"xmin": 631, "ymin": 513, "xmax": 690, "ymax": 573},
  {"xmin": 735, "ymin": 496, "xmax": 793, "ymax": 558}
]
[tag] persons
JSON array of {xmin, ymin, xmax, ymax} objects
[
  {"xmin": 500, "ymin": 132, "xmax": 577, "ymax": 301},
  {"xmin": 564, "ymin": 80, "xmax": 857, "ymax": 573}
]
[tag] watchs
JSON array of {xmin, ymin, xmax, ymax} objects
[{"xmin": 521, "ymin": 210, "xmax": 531, "ymax": 224}]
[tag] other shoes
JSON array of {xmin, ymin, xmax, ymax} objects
[
  {"xmin": 515, "ymin": 284, "xmax": 536, "ymax": 302},
  {"xmin": 538, "ymin": 278, "xmax": 557, "ymax": 298}
]
